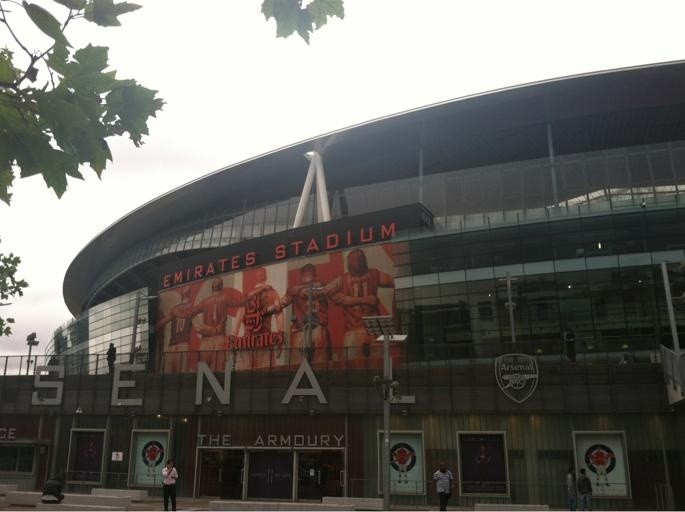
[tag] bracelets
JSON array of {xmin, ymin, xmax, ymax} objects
[{"xmin": 213, "ymin": 326, "xmax": 217, "ymax": 336}]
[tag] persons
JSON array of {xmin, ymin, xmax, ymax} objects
[
  {"xmin": 432, "ymin": 460, "xmax": 456, "ymax": 511},
  {"xmin": 251, "ymin": 262, "xmax": 377, "ymax": 366},
  {"xmin": 283, "ymin": 248, "xmax": 398, "ymax": 361},
  {"xmin": 155, "ymin": 284, "xmax": 225, "ymax": 374},
  {"xmin": 170, "ymin": 277, "xmax": 274, "ymax": 370},
  {"xmin": 48, "ymin": 355, "xmax": 61, "ymax": 376},
  {"xmin": 575, "ymin": 468, "xmax": 593, "ymax": 511},
  {"xmin": 40, "ymin": 473, "xmax": 66, "ymax": 504},
  {"xmin": 227, "ymin": 267, "xmax": 288, "ymax": 371},
  {"xmin": 106, "ymin": 342, "xmax": 117, "ymax": 375},
  {"xmin": 475, "ymin": 443, "xmax": 493, "ymax": 487},
  {"xmin": 161, "ymin": 459, "xmax": 180, "ymax": 511},
  {"xmin": 565, "ymin": 468, "xmax": 577, "ymax": 511}
]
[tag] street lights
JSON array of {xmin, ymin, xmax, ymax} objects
[
  {"xmin": 24, "ymin": 332, "xmax": 39, "ymax": 375},
  {"xmin": 361, "ymin": 313, "xmax": 399, "ymax": 511},
  {"xmin": 498, "ymin": 271, "xmax": 519, "ymax": 349}
]
